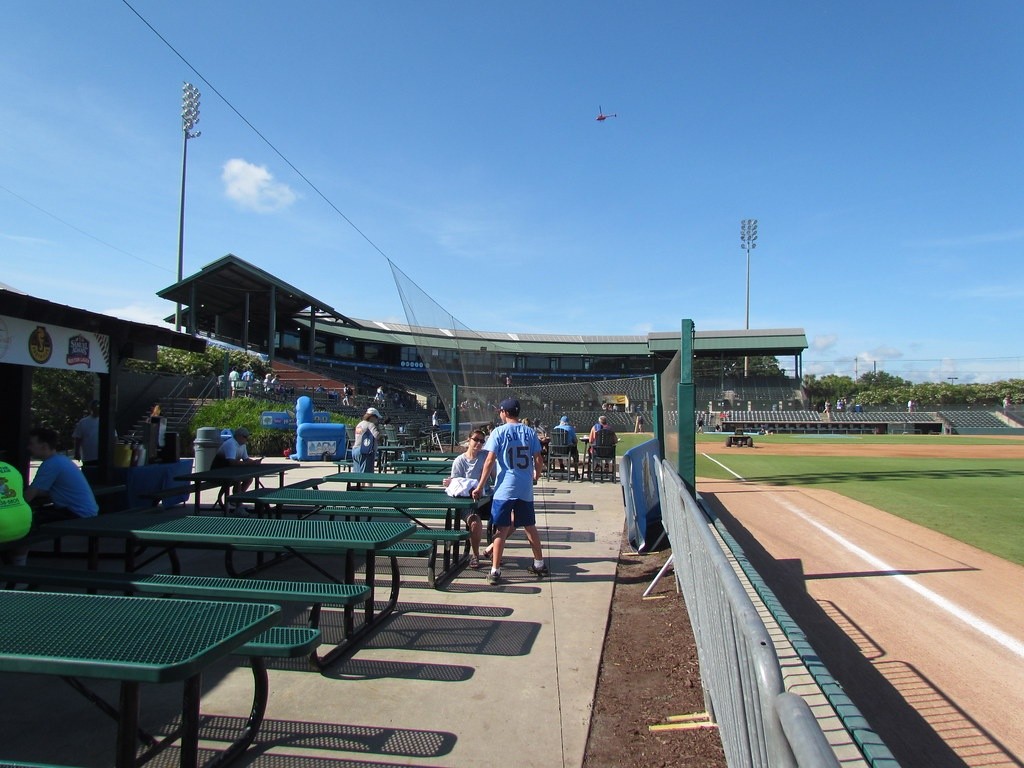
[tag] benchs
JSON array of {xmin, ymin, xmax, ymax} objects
[{"xmin": 0, "ymin": 460, "xmax": 472, "ymax": 768}]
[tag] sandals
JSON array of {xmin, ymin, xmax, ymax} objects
[
  {"xmin": 470, "ymin": 556, "xmax": 479, "ymax": 568},
  {"xmin": 484, "ymin": 550, "xmax": 494, "ymax": 559}
]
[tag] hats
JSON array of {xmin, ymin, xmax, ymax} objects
[
  {"xmin": 366, "ymin": 407, "xmax": 383, "ymax": 419},
  {"xmin": 497, "ymin": 398, "xmax": 520, "ymax": 417},
  {"xmin": 561, "ymin": 416, "xmax": 568, "ymax": 422},
  {"xmin": 236, "ymin": 427, "xmax": 252, "ymax": 437}
]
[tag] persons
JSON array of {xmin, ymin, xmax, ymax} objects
[
  {"xmin": 442, "ymin": 429, "xmax": 516, "ymax": 569},
  {"xmin": 23, "ymin": 428, "xmax": 99, "ymax": 530},
  {"xmin": 0, "ymin": 461, "xmax": 32, "ymax": 590},
  {"xmin": 553, "ymin": 416, "xmax": 580, "ymax": 479},
  {"xmin": 352, "ymin": 407, "xmax": 383, "ymax": 487},
  {"xmin": 71, "ymin": 400, "xmax": 119, "ymax": 486},
  {"xmin": 696, "ymin": 419, "xmax": 704, "ymax": 435},
  {"xmin": 822, "ymin": 399, "xmax": 831, "ymax": 413},
  {"xmin": 229, "ymin": 367, "xmax": 280, "ymax": 398},
  {"xmin": 588, "ymin": 416, "xmax": 618, "ymax": 472},
  {"xmin": 506, "ymin": 377, "xmax": 511, "ymax": 387},
  {"xmin": 432, "ymin": 411, "xmax": 439, "ymax": 428},
  {"xmin": 343, "ymin": 384, "xmax": 351, "ymax": 406},
  {"xmin": 639, "ymin": 419, "xmax": 643, "ymax": 432},
  {"xmin": 150, "ymin": 405, "xmax": 164, "ymax": 417},
  {"xmin": 316, "ymin": 384, "xmax": 325, "ymax": 392},
  {"xmin": 602, "ymin": 403, "xmax": 618, "ymax": 412},
  {"xmin": 472, "ymin": 398, "xmax": 548, "ymax": 585},
  {"xmin": 209, "ymin": 427, "xmax": 261, "ymax": 509},
  {"xmin": 374, "ymin": 385, "xmax": 384, "ymax": 407}
]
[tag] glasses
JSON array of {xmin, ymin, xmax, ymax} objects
[{"xmin": 470, "ymin": 437, "xmax": 485, "ymax": 444}]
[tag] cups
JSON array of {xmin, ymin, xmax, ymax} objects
[{"xmin": 402, "ymin": 451, "xmax": 408, "ymax": 461}]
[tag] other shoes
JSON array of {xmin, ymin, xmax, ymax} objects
[
  {"xmin": 594, "ymin": 464, "xmax": 601, "ymax": 471},
  {"xmin": 603, "ymin": 463, "xmax": 610, "ymax": 473},
  {"xmin": 575, "ymin": 473, "xmax": 580, "ymax": 478}
]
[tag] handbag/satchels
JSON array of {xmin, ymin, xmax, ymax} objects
[{"xmin": 360, "ymin": 429, "xmax": 375, "ymax": 454}]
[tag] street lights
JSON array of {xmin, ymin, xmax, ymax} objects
[
  {"xmin": 947, "ymin": 377, "xmax": 958, "ymax": 385},
  {"xmin": 173, "ymin": 82, "xmax": 202, "ymax": 332},
  {"xmin": 738, "ymin": 218, "xmax": 759, "ymax": 410}
]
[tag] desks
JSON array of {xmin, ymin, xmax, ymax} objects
[
  {"xmin": 408, "ymin": 452, "xmax": 461, "ymax": 461},
  {"xmin": 580, "ymin": 438, "xmax": 620, "ymax": 482},
  {"xmin": 0, "ymin": 590, "xmax": 286, "ymax": 768},
  {"xmin": 174, "ymin": 464, "xmax": 300, "ymax": 517},
  {"xmin": 85, "ymin": 457, "xmax": 192, "ymax": 506},
  {"xmin": 227, "ymin": 488, "xmax": 475, "ymax": 576},
  {"xmin": 40, "ymin": 515, "xmax": 417, "ymax": 639},
  {"xmin": 324, "ymin": 473, "xmax": 451, "ymax": 491},
  {"xmin": 33, "ymin": 483, "xmax": 127, "ymax": 519},
  {"xmin": 381, "ymin": 461, "xmax": 453, "ymax": 474}
]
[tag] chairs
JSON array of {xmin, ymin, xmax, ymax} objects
[
  {"xmin": 588, "ymin": 429, "xmax": 616, "ymax": 484},
  {"xmin": 344, "ymin": 422, "xmax": 431, "ymax": 468},
  {"xmin": 547, "ymin": 428, "xmax": 577, "ymax": 482}
]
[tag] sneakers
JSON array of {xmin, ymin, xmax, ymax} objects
[
  {"xmin": 486, "ymin": 570, "xmax": 501, "ymax": 584},
  {"xmin": 526, "ymin": 563, "xmax": 549, "ymax": 576}
]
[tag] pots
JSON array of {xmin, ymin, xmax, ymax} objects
[{"xmin": 114, "ymin": 437, "xmax": 146, "ymax": 467}]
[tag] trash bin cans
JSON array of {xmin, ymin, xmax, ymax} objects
[
  {"xmin": 193, "ymin": 426, "xmax": 222, "ymax": 473},
  {"xmin": 856, "ymin": 405, "xmax": 861, "ymax": 412}
]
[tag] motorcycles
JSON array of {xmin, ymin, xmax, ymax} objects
[{"xmin": 725, "ymin": 428, "xmax": 753, "ymax": 448}]
